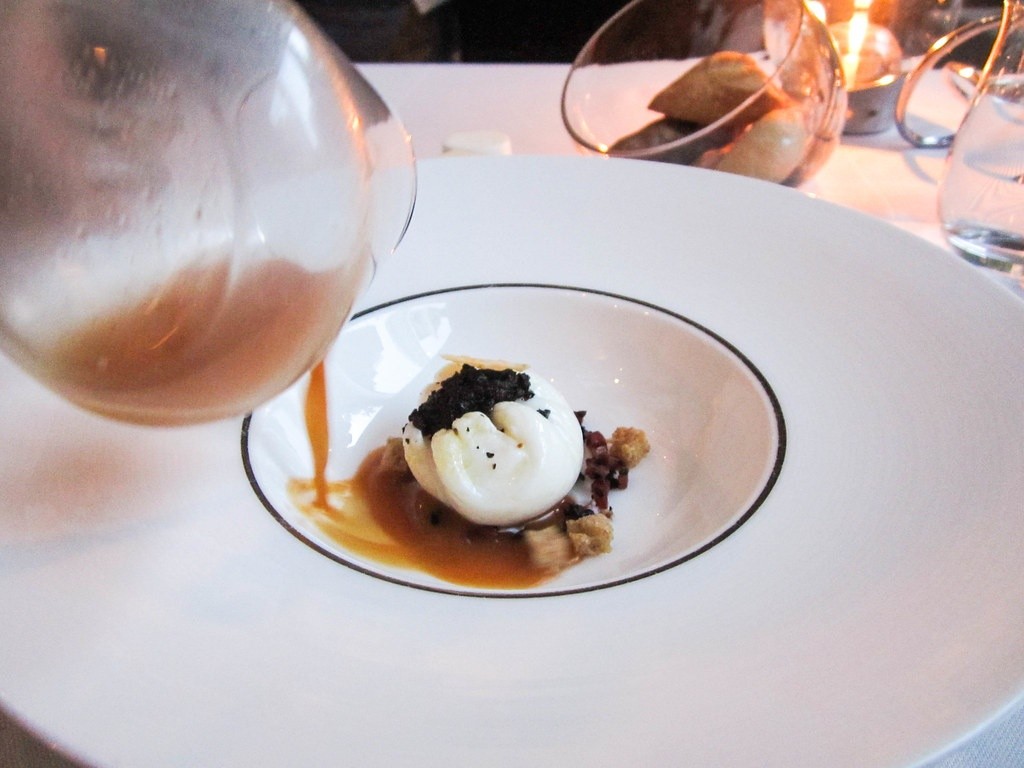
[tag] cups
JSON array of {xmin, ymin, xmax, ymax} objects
[
  {"xmin": 0, "ymin": 0, "xmax": 417, "ymax": 434},
  {"xmin": 938, "ymin": 0, "xmax": 1024, "ymax": 279}
]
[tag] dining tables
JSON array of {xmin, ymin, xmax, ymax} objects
[{"xmin": 0, "ymin": 56, "xmax": 1024, "ymax": 768}]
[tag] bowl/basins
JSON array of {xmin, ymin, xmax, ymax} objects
[{"xmin": 560, "ymin": 0, "xmax": 843, "ymax": 192}]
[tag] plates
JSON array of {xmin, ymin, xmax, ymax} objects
[{"xmin": 0, "ymin": 153, "xmax": 1024, "ymax": 768}]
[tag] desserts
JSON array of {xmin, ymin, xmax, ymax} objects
[{"xmin": 380, "ymin": 355, "xmax": 651, "ymax": 575}]
